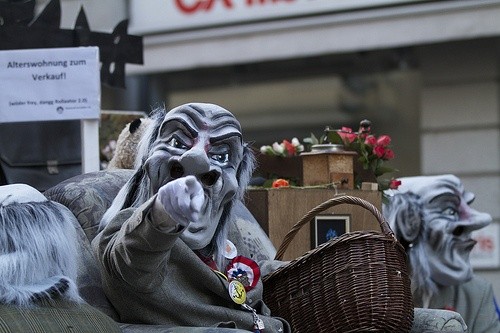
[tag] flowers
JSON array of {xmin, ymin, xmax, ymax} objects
[{"xmin": 260, "ymin": 126, "xmax": 402, "ymax": 206}]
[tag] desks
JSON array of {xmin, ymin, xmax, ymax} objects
[{"xmin": 245, "ymin": 188, "xmax": 382, "ymax": 261}]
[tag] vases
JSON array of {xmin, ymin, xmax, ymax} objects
[{"xmin": 289, "ymin": 156, "xmax": 355, "ymax": 188}]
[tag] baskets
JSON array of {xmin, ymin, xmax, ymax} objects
[{"xmin": 262, "ymin": 196, "xmax": 415, "ymax": 333}]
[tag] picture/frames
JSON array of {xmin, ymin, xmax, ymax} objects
[{"xmin": 310, "ymin": 213, "xmax": 351, "ymax": 250}]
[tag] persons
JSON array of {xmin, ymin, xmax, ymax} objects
[
  {"xmin": 99, "ymin": 103, "xmax": 290, "ymax": 333},
  {"xmin": 382, "ymin": 175, "xmax": 500, "ymax": 333},
  {"xmin": 0, "ymin": 184, "xmax": 122, "ymax": 333}
]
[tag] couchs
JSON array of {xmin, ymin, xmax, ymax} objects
[{"xmin": 42, "ymin": 169, "xmax": 468, "ymax": 333}]
